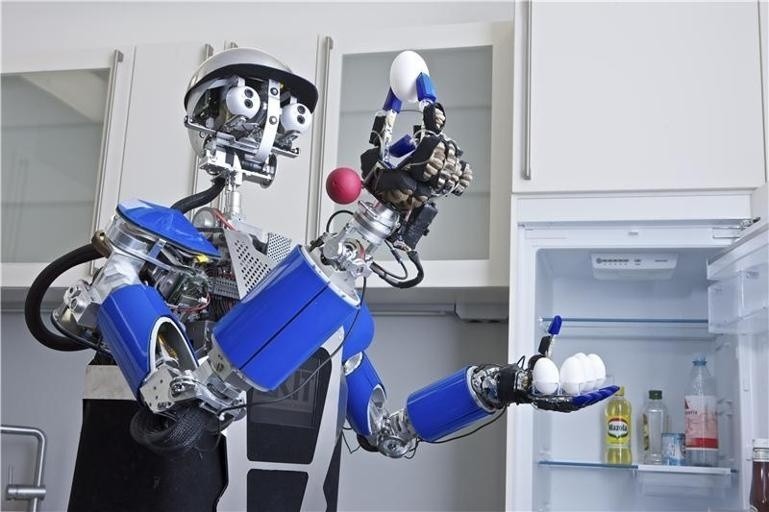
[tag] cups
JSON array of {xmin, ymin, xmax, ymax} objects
[{"xmin": 659, "ymin": 432, "xmax": 686, "ymax": 465}]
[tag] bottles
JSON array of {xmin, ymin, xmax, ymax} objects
[
  {"xmin": 684, "ymin": 355, "xmax": 720, "ymax": 468},
  {"xmin": 749, "ymin": 437, "xmax": 769, "ymax": 512},
  {"xmin": 605, "ymin": 386, "xmax": 633, "ymax": 465},
  {"xmin": 641, "ymin": 390, "xmax": 669, "ymax": 465}
]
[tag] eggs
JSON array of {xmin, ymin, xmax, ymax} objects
[
  {"xmin": 589, "ymin": 353, "xmax": 607, "ymax": 388},
  {"xmin": 389, "ymin": 49, "xmax": 429, "ymax": 105},
  {"xmin": 561, "ymin": 356, "xmax": 585, "ymax": 395},
  {"xmin": 576, "ymin": 351, "xmax": 594, "ymax": 392},
  {"xmin": 533, "ymin": 356, "xmax": 561, "ymax": 394}
]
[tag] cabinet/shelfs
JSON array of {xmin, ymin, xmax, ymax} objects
[
  {"xmin": 304, "ymin": 20, "xmax": 508, "ymax": 288},
  {"xmin": 511, "ymin": 0, "xmax": 766, "ymax": 196},
  {"xmin": 0, "ymin": 40, "xmax": 134, "ymax": 288},
  {"xmin": 119, "ymin": 34, "xmax": 319, "ymax": 252}
]
